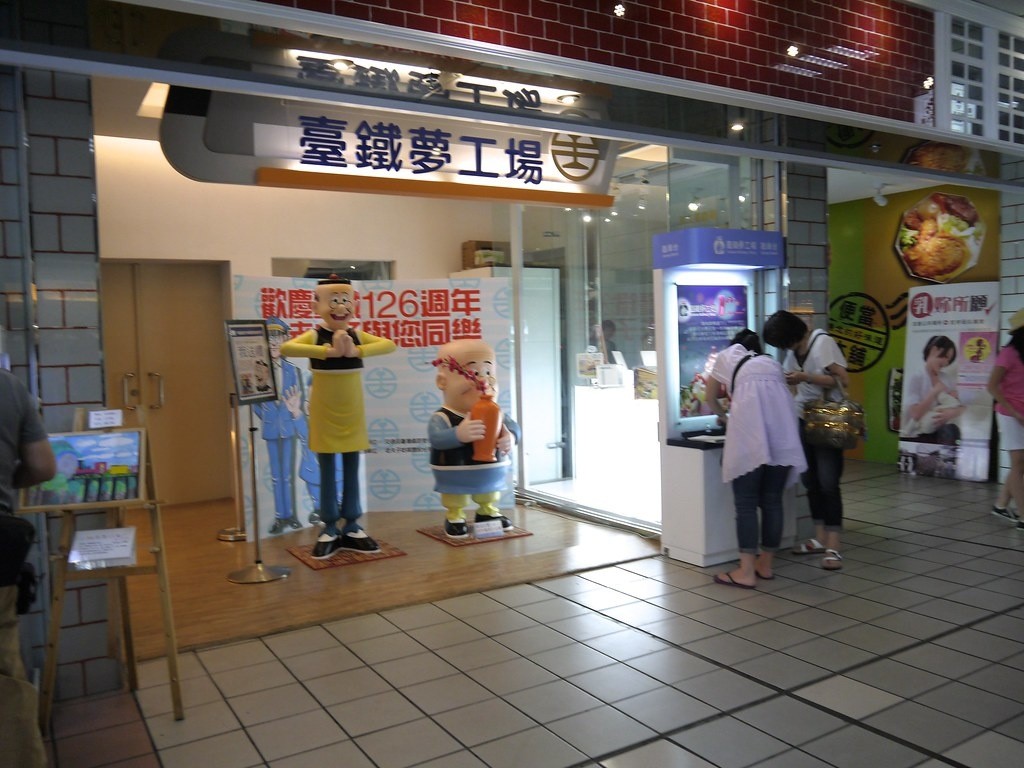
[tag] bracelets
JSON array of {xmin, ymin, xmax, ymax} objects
[{"xmin": 807, "ymin": 371, "xmax": 813, "ymax": 384}]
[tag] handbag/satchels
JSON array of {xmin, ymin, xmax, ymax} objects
[{"xmin": 804, "ymin": 373, "xmax": 865, "ymax": 449}]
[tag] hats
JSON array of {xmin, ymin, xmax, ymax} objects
[
  {"xmin": 957, "ymin": 386, "xmax": 982, "ymax": 405},
  {"xmin": 1008, "ymin": 308, "xmax": 1024, "ymax": 334}
]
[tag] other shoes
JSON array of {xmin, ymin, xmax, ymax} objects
[
  {"xmin": 1016, "ymin": 520, "xmax": 1024, "ymax": 531},
  {"xmin": 991, "ymin": 505, "xmax": 1022, "ymax": 522}
]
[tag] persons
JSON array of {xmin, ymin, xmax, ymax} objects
[
  {"xmin": 904, "ymin": 335, "xmax": 970, "ymax": 445},
  {"xmin": 987, "ymin": 325, "xmax": 1024, "ymax": 530},
  {"xmin": 426, "ymin": 340, "xmax": 522, "ymax": 539},
  {"xmin": 704, "ymin": 329, "xmax": 808, "ymax": 589},
  {"xmin": 919, "ymin": 390, "xmax": 975, "ymax": 434},
  {"xmin": 762, "ymin": 310, "xmax": 865, "ymax": 570},
  {"xmin": 602, "ymin": 320, "xmax": 616, "ymax": 364},
  {"xmin": 278, "ymin": 275, "xmax": 395, "ymax": 558},
  {"xmin": 0, "ymin": 367, "xmax": 55, "ymax": 768}
]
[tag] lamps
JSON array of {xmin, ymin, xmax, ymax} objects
[
  {"xmin": 689, "ymin": 197, "xmax": 701, "ymax": 211},
  {"xmin": 738, "ymin": 188, "xmax": 748, "ymax": 202},
  {"xmin": 872, "ymin": 184, "xmax": 888, "ymax": 207}
]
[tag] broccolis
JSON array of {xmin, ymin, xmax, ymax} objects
[{"xmin": 899, "ymin": 226, "xmax": 920, "ymax": 250}]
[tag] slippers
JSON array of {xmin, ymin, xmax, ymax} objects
[
  {"xmin": 714, "ymin": 572, "xmax": 757, "ymax": 590},
  {"xmin": 821, "ymin": 548, "xmax": 843, "ymax": 570},
  {"xmin": 792, "ymin": 539, "xmax": 825, "ymax": 554},
  {"xmin": 754, "ymin": 570, "xmax": 774, "ymax": 580}
]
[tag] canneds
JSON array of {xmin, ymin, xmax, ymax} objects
[{"xmin": 27, "ymin": 475, "xmax": 138, "ymax": 508}]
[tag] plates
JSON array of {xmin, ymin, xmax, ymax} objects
[{"xmin": 895, "ymin": 192, "xmax": 987, "ymax": 284}]
